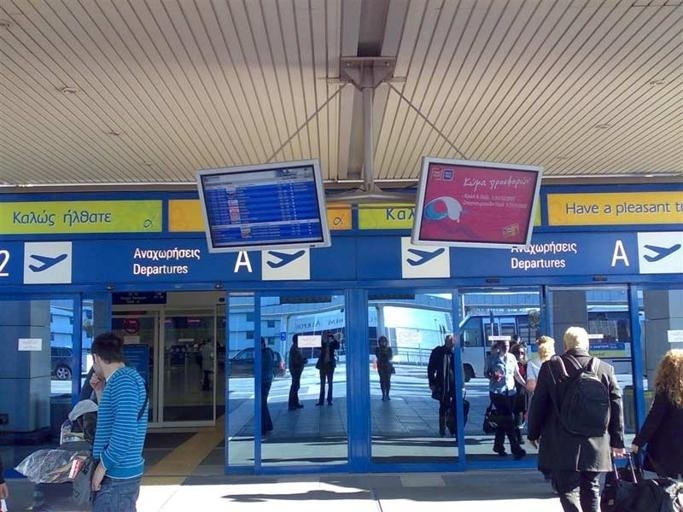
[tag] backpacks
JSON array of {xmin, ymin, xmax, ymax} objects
[{"xmin": 545, "ymin": 351, "xmax": 613, "ymax": 438}]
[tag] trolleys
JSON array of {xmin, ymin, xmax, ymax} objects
[{"xmin": 25, "ymin": 403, "xmax": 99, "ymax": 512}]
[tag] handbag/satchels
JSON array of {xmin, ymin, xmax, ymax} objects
[
  {"xmin": 483, "ymin": 408, "xmax": 514, "ymax": 434},
  {"xmin": 72, "ymin": 456, "xmax": 96, "ymax": 512},
  {"xmin": 60, "ymin": 417, "xmax": 83, "ymax": 445},
  {"xmin": 599, "ymin": 468, "xmax": 643, "ymax": 511}
]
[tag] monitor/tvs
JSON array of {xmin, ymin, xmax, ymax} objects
[
  {"xmin": 196, "ymin": 160, "xmax": 331, "ymax": 252},
  {"xmin": 410, "ymin": 157, "xmax": 544, "ymax": 251}
]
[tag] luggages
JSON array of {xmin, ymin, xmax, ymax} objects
[{"xmin": 446, "ymin": 389, "xmax": 468, "ymax": 437}]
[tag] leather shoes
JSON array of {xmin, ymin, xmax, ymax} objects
[
  {"xmin": 493, "ymin": 448, "xmax": 507, "ymax": 456},
  {"xmin": 513, "ymin": 450, "xmax": 525, "ymax": 461}
]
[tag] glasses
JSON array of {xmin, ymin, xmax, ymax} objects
[{"xmin": 515, "ymin": 350, "xmax": 525, "ymax": 356}]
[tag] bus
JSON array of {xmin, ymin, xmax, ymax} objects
[{"xmin": 451, "ymin": 308, "xmax": 644, "ymax": 377}]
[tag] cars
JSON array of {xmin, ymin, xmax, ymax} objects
[{"xmin": 230, "ymin": 347, "xmax": 290, "ymax": 380}]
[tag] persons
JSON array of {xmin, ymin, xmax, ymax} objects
[
  {"xmin": 375, "ymin": 335, "xmax": 395, "ymax": 400},
  {"xmin": 316, "ymin": 332, "xmax": 340, "ymax": 406},
  {"xmin": 485, "ymin": 334, "xmax": 527, "ymax": 460},
  {"xmin": 0, "ymin": 457, "xmax": 9, "ymax": 500},
  {"xmin": 630, "ymin": 349, "xmax": 683, "ymax": 479},
  {"xmin": 76, "ymin": 366, "xmax": 98, "ymax": 445},
  {"xmin": 526, "ymin": 336, "xmax": 558, "ymax": 402},
  {"xmin": 92, "ymin": 333, "xmax": 150, "ymax": 511},
  {"xmin": 288, "ymin": 334, "xmax": 308, "ymax": 408},
  {"xmin": 200, "ymin": 336, "xmax": 214, "ymax": 391},
  {"xmin": 428, "ymin": 335, "xmax": 465, "ymax": 437},
  {"xmin": 254, "ymin": 337, "xmax": 274, "ymax": 435},
  {"xmin": 528, "ymin": 327, "xmax": 627, "ymax": 512}
]
